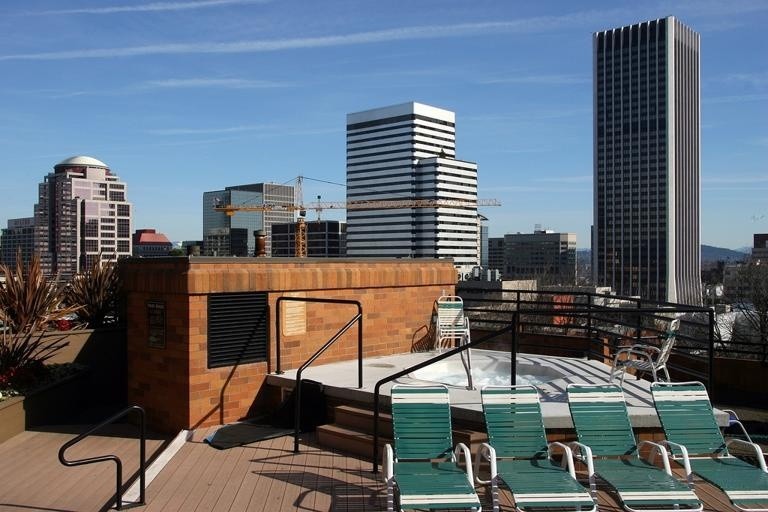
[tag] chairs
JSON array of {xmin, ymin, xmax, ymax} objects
[
  {"xmin": 607, "ymin": 311, "xmax": 683, "ymax": 393},
  {"xmin": 428, "ymin": 291, "xmax": 475, "ymax": 363},
  {"xmin": 647, "ymin": 380, "xmax": 768, "ymax": 512},
  {"xmin": 475, "ymin": 385, "xmax": 599, "ymax": 511},
  {"xmin": 382, "ymin": 383, "xmax": 482, "ymax": 511},
  {"xmin": 566, "ymin": 383, "xmax": 705, "ymax": 509}
]
